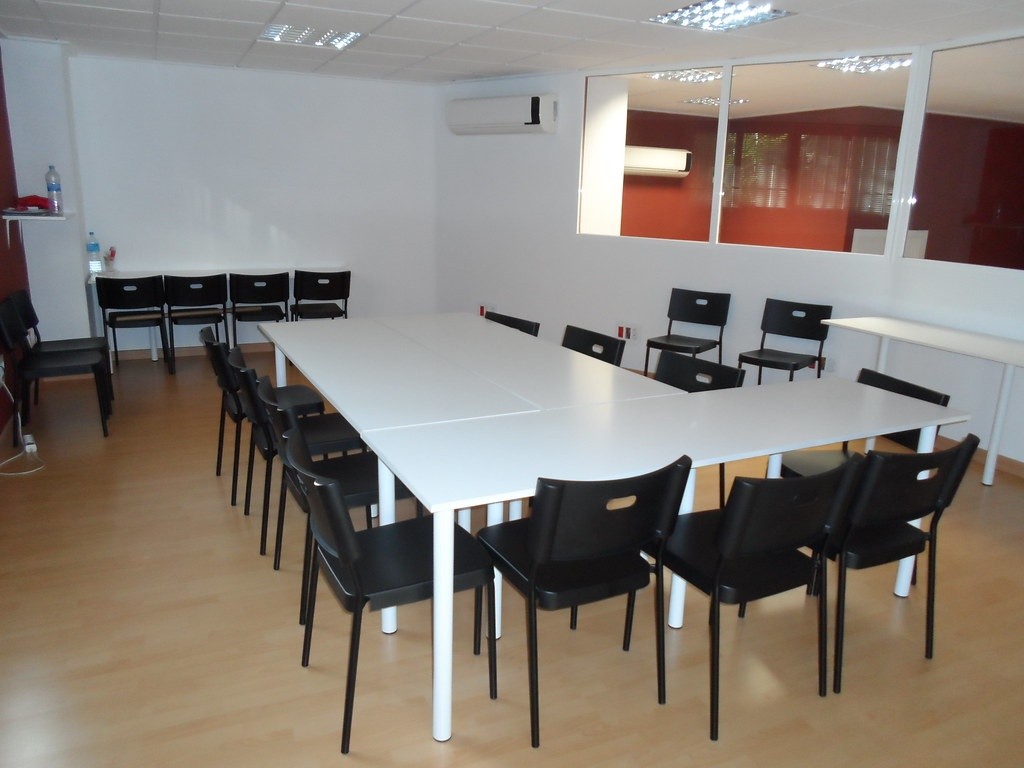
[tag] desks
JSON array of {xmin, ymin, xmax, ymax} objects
[
  {"xmin": 360, "ymin": 377, "xmax": 968, "ymax": 742},
  {"xmin": 87, "ymin": 271, "xmax": 342, "ymax": 374},
  {"xmin": 256, "ymin": 319, "xmax": 542, "ymax": 634},
  {"xmin": 373, "ymin": 312, "xmax": 688, "ymax": 521},
  {"xmin": 820, "ymin": 315, "xmax": 1024, "ymax": 487}
]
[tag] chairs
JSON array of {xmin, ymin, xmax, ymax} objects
[
  {"xmin": 0, "ymin": 298, "xmax": 110, "ymax": 438},
  {"xmin": 229, "ymin": 271, "xmax": 290, "ymax": 348},
  {"xmin": 291, "ymin": 270, "xmax": 351, "ymax": 367},
  {"xmin": 227, "ymin": 347, "xmax": 371, "ymax": 556},
  {"xmin": 484, "ymin": 287, "xmax": 835, "ymax": 393},
  {"xmin": 256, "ymin": 372, "xmax": 424, "ymax": 624},
  {"xmin": 474, "ymin": 455, "xmax": 692, "ymax": 746},
  {"xmin": 619, "ymin": 452, "xmax": 865, "ymax": 742},
  {"xmin": 164, "ymin": 273, "xmax": 230, "ymax": 375},
  {"xmin": 96, "ymin": 274, "xmax": 172, "ymax": 377},
  {"xmin": 805, "ymin": 433, "xmax": 979, "ymax": 693},
  {"xmin": 198, "ymin": 325, "xmax": 326, "ymax": 507},
  {"xmin": 277, "ymin": 427, "xmax": 498, "ymax": 755},
  {"xmin": 7, "ymin": 289, "xmax": 115, "ymax": 415}
]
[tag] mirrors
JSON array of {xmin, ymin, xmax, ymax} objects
[
  {"xmin": 577, "ymin": 52, "xmax": 723, "ymax": 242},
  {"xmin": 902, "ymin": 35, "xmax": 1024, "ymax": 270},
  {"xmin": 716, "ymin": 54, "xmax": 913, "ymax": 255}
]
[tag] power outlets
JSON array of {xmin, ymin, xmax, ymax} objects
[{"xmin": 0, "ymin": 354, "xmax": 5, "ymax": 388}]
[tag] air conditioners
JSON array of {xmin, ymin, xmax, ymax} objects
[
  {"xmin": 624, "ymin": 145, "xmax": 694, "ymax": 179},
  {"xmin": 444, "ymin": 93, "xmax": 558, "ymax": 135}
]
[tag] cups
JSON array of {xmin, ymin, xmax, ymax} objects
[{"xmin": 105, "ymin": 261, "xmax": 113, "ymax": 272}]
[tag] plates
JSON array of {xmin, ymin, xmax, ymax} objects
[{"xmin": 2, "ymin": 207, "xmax": 48, "ymax": 215}]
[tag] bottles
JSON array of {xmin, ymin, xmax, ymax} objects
[
  {"xmin": 85, "ymin": 232, "xmax": 104, "ymax": 277},
  {"xmin": 44, "ymin": 165, "xmax": 64, "ymax": 216}
]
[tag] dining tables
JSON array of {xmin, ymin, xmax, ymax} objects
[{"xmin": 764, "ymin": 367, "xmax": 951, "ymax": 598}]
